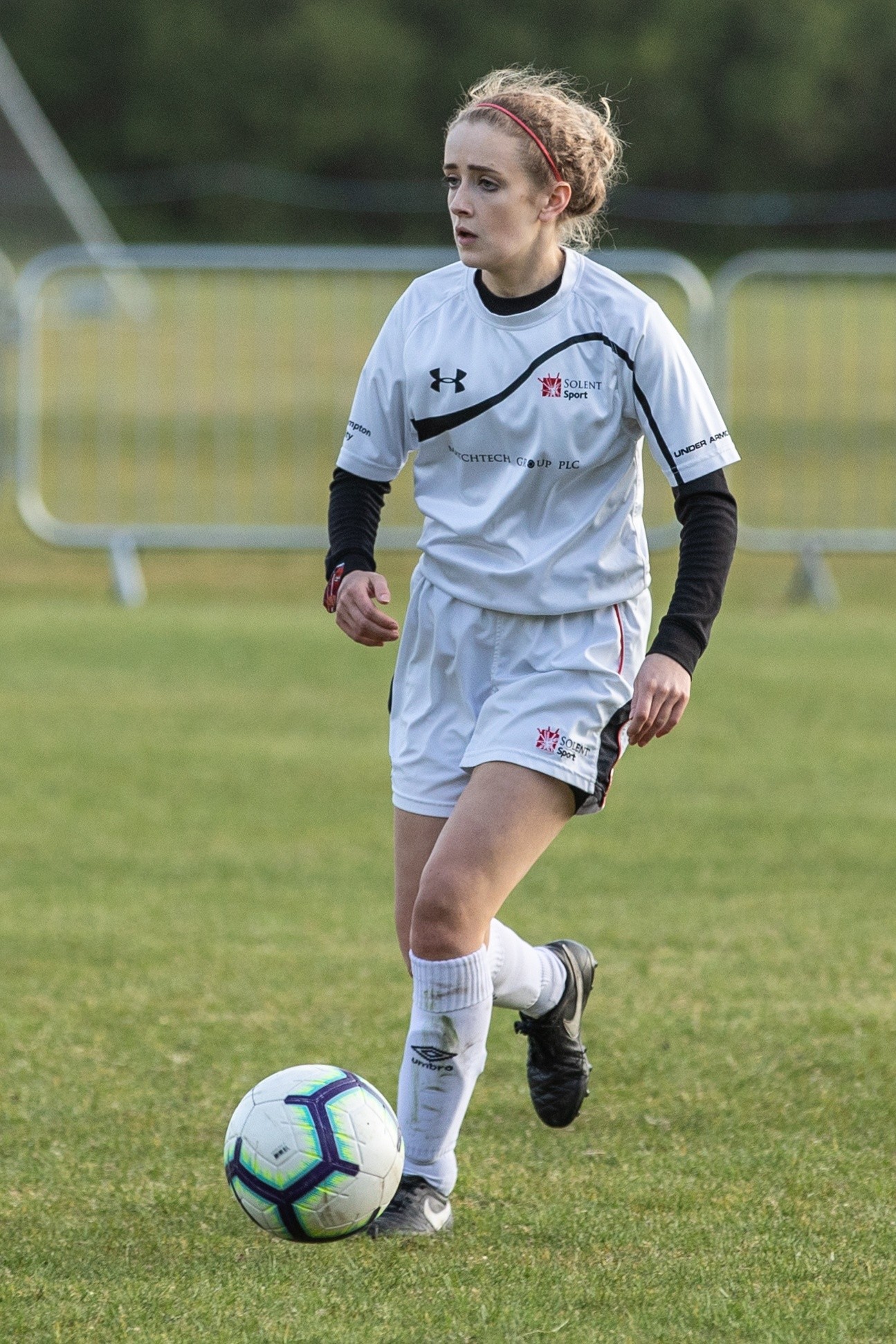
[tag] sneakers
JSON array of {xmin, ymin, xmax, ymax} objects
[
  {"xmin": 365, "ymin": 1173, "xmax": 453, "ymax": 1238},
  {"xmin": 514, "ymin": 940, "xmax": 600, "ymax": 1129}
]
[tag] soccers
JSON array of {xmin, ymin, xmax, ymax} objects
[{"xmin": 223, "ymin": 1063, "xmax": 405, "ymax": 1244}]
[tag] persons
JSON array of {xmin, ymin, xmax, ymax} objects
[{"xmin": 322, "ymin": 71, "xmax": 747, "ymax": 1238}]
